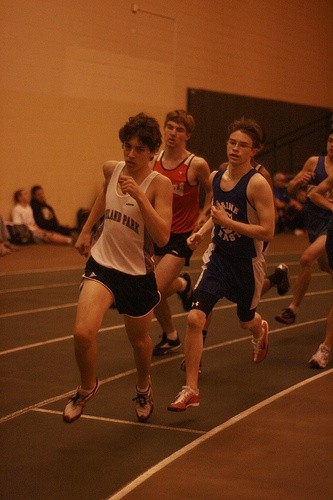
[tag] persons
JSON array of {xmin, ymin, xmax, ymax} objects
[
  {"xmin": 150, "ymin": 110, "xmax": 214, "ymax": 356},
  {"xmin": 308, "ymin": 173, "xmax": 333, "ymax": 366},
  {"xmin": 274, "ymin": 130, "xmax": 333, "ymax": 325},
  {"xmin": 178, "ymin": 142, "xmax": 290, "ymax": 372},
  {"xmin": 63, "ymin": 111, "xmax": 174, "ymax": 424},
  {"xmin": 167, "ymin": 118, "xmax": 269, "ymax": 412},
  {"xmin": 0, "ymin": 184, "xmax": 81, "ymax": 256},
  {"xmin": 270, "ymin": 172, "xmax": 309, "ymax": 232}
]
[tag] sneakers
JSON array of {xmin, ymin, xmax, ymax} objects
[
  {"xmin": 166, "ymin": 385, "xmax": 199, "ymax": 413},
  {"xmin": 179, "ymin": 358, "xmax": 201, "ymax": 375},
  {"xmin": 276, "ymin": 263, "xmax": 289, "ymax": 296},
  {"xmin": 309, "ymin": 343, "xmax": 331, "ymax": 368},
  {"xmin": 176, "ymin": 271, "xmax": 193, "ymax": 312},
  {"xmin": 273, "ymin": 307, "xmax": 295, "ymax": 324},
  {"xmin": 151, "ymin": 331, "xmax": 180, "ymax": 356},
  {"xmin": 134, "ymin": 376, "xmax": 153, "ymax": 423},
  {"xmin": 250, "ymin": 320, "xmax": 268, "ymax": 364},
  {"xmin": 62, "ymin": 378, "xmax": 100, "ymax": 424}
]
